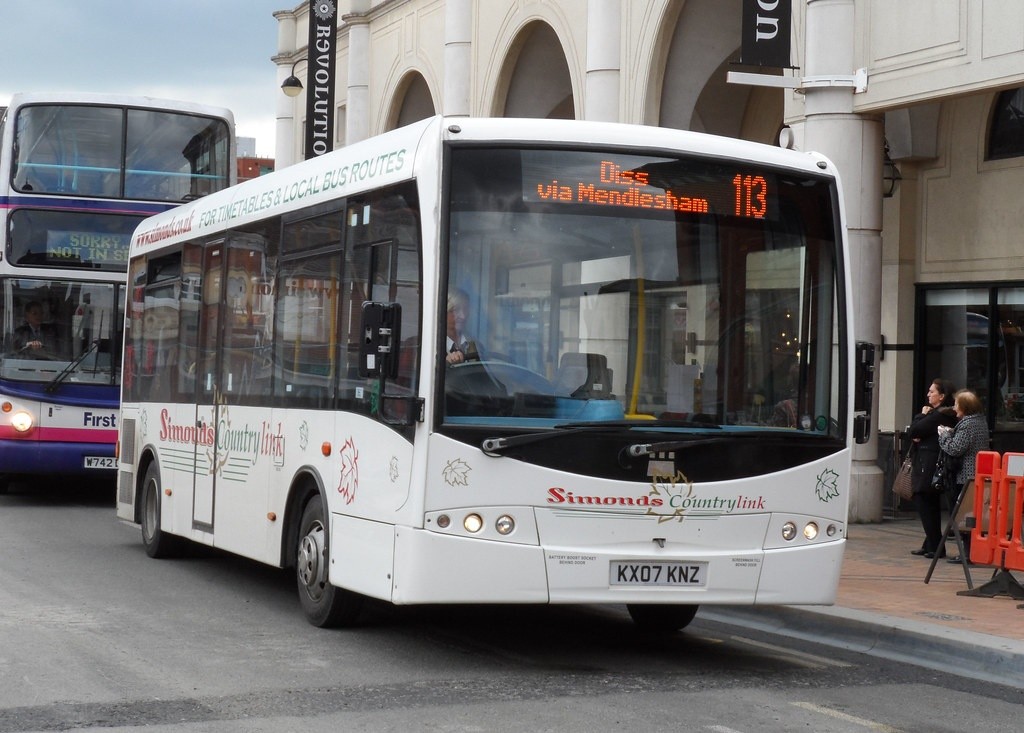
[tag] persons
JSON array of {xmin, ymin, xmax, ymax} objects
[
  {"xmin": 445, "ymin": 285, "xmax": 489, "ymax": 365},
  {"xmin": 907, "ymin": 378, "xmax": 990, "ymax": 565},
  {"xmin": 14, "ymin": 300, "xmax": 62, "ymax": 353}
]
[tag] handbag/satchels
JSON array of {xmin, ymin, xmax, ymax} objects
[
  {"xmin": 893, "ymin": 442, "xmax": 916, "ymax": 501},
  {"xmin": 931, "ymin": 450, "xmax": 954, "ymax": 492}
]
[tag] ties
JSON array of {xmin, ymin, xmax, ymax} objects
[{"xmin": 35, "ymin": 329, "xmax": 39, "ymax": 339}]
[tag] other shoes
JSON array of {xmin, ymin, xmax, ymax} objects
[
  {"xmin": 947, "ymin": 555, "xmax": 971, "ymax": 564},
  {"xmin": 925, "ymin": 552, "xmax": 946, "ymax": 558},
  {"xmin": 946, "ymin": 529, "xmax": 955, "ymax": 537},
  {"xmin": 910, "ymin": 548, "xmax": 925, "ymax": 554}
]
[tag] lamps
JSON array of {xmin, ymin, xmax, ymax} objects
[
  {"xmin": 879, "ymin": 146, "xmax": 901, "ymax": 196},
  {"xmin": 281, "ymin": 56, "xmax": 306, "ymax": 97}
]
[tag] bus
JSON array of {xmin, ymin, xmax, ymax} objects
[
  {"xmin": 112, "ymin": 119, "xmax": 860, "ymax": 636},
  {"xmin": 1, "ymin": 91, "xmax": 240, "ymax": 510}
]
